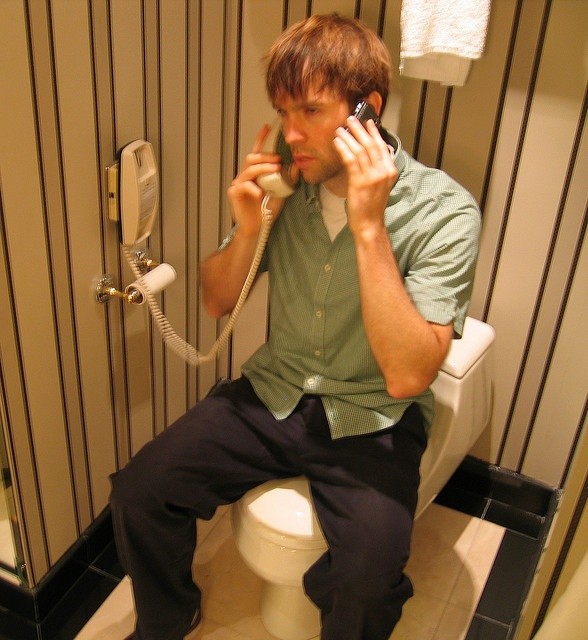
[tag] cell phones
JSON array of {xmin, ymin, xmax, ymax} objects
[{"xmin": 345, "ymin": 100, "xmax": 381, "ymax": 138}]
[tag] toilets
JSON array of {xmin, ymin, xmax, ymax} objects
[{"xmin": 231, "ymin": 316, "xmax": 497, "ymax": 640}]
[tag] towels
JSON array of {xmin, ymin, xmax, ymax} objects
[{"xmin": 399, "ymin": 2, "xmax": 492, "ymax": 88}]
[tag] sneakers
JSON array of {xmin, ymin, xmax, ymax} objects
[{"xmin": 124, "ymin": 608, "xmax": 202, "ymax": 640}]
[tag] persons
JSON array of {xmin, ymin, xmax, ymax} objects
[{"xmin": 109, "ymin": 11, "xmax": 483, "ymax": 640}]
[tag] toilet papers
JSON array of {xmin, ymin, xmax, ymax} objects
[{"xmin": 124, "ymin": 262, "xmax": 177, "ymax": 306}]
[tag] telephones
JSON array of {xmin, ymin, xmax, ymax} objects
[{"xmin": 250, "ymin": 115, "xmax": 294, "ymax": 200}]
